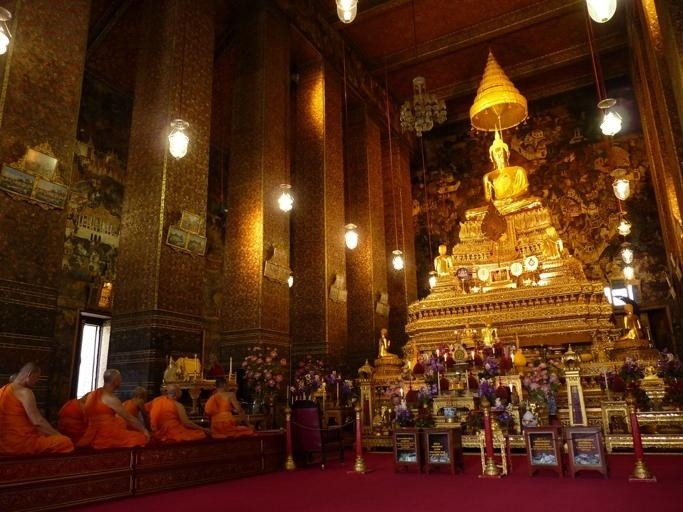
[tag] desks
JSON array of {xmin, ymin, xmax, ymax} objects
[
  {"xmin": 565, "ymin": 426, "xmax": 611, "ymax": 481},
  {"xmin": 392, "ymin": 428, "xmax": 427, "ymax": 475},
  {"xmin": 160, "ymin": 380, "xmax": 240, "ymax": 415},
  {"xmin": 524, "ymin": 426, "xmax": 563, "ymax": 478},
  {"xmin": 326, "ymin": 407, "xmax": 354, "ymax": 439},
  {"xmin": 423, "ymin": 427, "xmax": 464, "ymax": 474}
]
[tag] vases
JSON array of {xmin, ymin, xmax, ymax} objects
[{"xmin": 253, "ymin": 395, "xmax": 271, "ymax": 426}]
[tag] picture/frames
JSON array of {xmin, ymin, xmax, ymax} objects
[
  {"xmin": 0, "ymin": 147, "xmax": 68, "ymax": 210},
  {"xmin": 263, "ymin": 247, "xmax": 292, "ymax": 283},
  {"xmin": 329, "ymin": 274, "xmax": 348, "ymax": 303},
  {"xmin": 376, "ymin": 290, "xmax": 391, "ymax": 317},
  {"xmin": 166, "ymin": 210, "xmax": 208, "ymax": 256}
]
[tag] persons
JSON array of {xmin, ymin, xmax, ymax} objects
[
  {"xmin": 84, "ymin": 367, "xmax": 148, "ymax": 448},
  {"xmin": 544, "ymin": 225, "xmax": 563, "ymax": 260},
  {"xmin": 378, "ymin": 328, "xmax": 397, "ymax": 357},
  {"xmin": 114, "ymin": 386, "xmax": 149, "ymax": 430},
  {"xmin": 434, "ymin": 243, "xmax": 453, "ymax": 276},
  {"xmin": 58, "ymin": 391, "xmax": 91, "ymax": 446},
  {"xmin": 204, "ymin": 378, "xmax": 257, "ymax": 438},
  {"xmin": 620, "ymin": 305, "xmax": 641, "ymax": 339},
  {"xmin": 149, "ymin": 383, "xmax": 209, "ymax": 442},
  {"xmin": 0, "ymin": 362, "xmax": 75, "ymax": 455},
  {"xmin": 9, "ymin": 372, "xmax": 18, "ymax": 383}
]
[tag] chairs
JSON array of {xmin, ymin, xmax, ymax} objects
[{"xmin": 291, "ymin": 400, "xmax": 346, "ymax": 469}]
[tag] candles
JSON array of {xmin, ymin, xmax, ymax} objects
[
  {"xmin": 194, "ymin": 353, "xmax": 197, "ymax": 370},
  {"xmin": 291, "ymin": 381, "xmax": 339, "ymax": 409},
  {"xmin": 230, "ymin": 357, "xmax": 233, "ymax": 373},
  {"xmin": 604, "ymin": 370, "xmax": 608, "ymax": 388},
  {"xmin": 399, "ymin": 349, "xmax": 515, "ymax": 398}
]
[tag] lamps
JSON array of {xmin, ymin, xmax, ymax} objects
[
  {"xmin": 384, "ymin": 64, "xmax": 404, "ymax": 271},
  {"xmin": 586, "ymin": 1, "xmax": 634, "ymax": 281},
  {"xmin": 167, "ymin": 1, "xmax": 189, "ymax": 158},
  {"xmin": 0, "ymin": 7, "xmax": 12, "ymax": 54},
  {"xmin": 399, "ymin": 0, "xmax": 448, "ymax": 137},
  {"xmin": 343, "ymin": 36, "xmax": 360, "ymax": 251},
  {"xmin": 276, "ymin": 17, "xmax": 295, "ymax": 214},
  {"xmin": 336, "ymin": 0, "xmax": 358, "ymax": 24},
  {"xmin": 420, "ymin": 136, "xmax": 437, "ymax": 288}
]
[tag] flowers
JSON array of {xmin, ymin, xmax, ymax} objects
[{"xmin": 240, "ymin": 345, "xmax": 286, "ymax": 397}]
